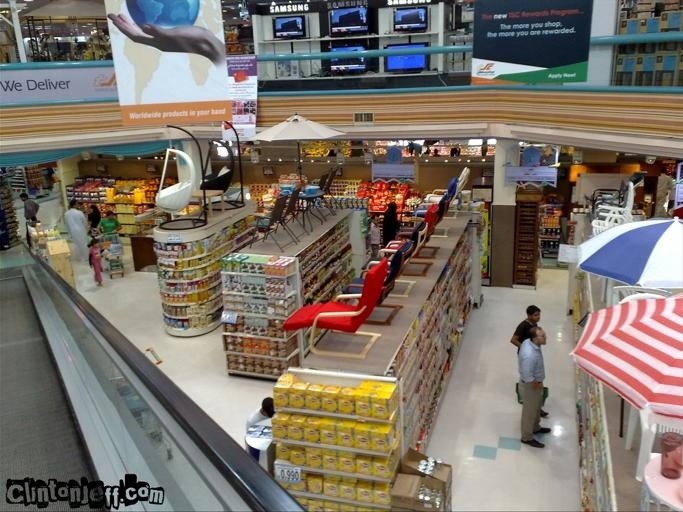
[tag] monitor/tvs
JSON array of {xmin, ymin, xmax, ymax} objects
[
  {"xmin": 384, "ymin": 42, "xmax": 429, "ymax": 72},
  {"xmin": 391, "ymin": 6, "xmax": 429, "ymax": 33},
  {"xmin": 329, "ymin": 46, "xmax": 368, "ymax": 74},
  {"xmin": 273, "ymin": 15, "xmax": 306, "ymax": 39},
  {"xmin": 330, "ymin": 8, "xmax": 371, "ymax": 36}
]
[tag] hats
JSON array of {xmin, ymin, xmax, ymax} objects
[{"xmin": 262, "ymin": 398, "xmax": 273, "ymax": 418}]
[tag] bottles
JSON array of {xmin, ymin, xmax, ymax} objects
[{"xmin": 659, "ymin": 429, "xmax": 682, "ymax": 479}]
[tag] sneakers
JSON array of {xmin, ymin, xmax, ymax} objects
[{"xmin": 540, "ymin": 408, "xmax": 548, "ymax": 416}]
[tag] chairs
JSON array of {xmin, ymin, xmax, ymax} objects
[{"xmin": 154, "ymin": 121, "xmax": 245, "ymax": 230}]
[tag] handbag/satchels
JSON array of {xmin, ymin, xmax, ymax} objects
[{"xmin": 516, "ymin": 383, "xmax": 548, "ymax": 404}]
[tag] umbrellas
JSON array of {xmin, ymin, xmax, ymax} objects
[
  {"xmin": 250, "ymin": 112, "xmax": 346, "ymax": 179},
  {"xmin": 575, "ymin": 216, "xmax": 683, "ymax": 293},
  {"xmin": 569, "ymin": 294, "xmax": 683, "ymax": 429}
]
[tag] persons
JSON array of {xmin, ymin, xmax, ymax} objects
[
  {"xmin": 106, "ymin": 12, "xmax": 225, "ymax": 66},
  {"xmin": 367, "ymin": 215, "xmax": 380, "ymax": 256},
  {"xmin": 86, "ymin": 204, "xmax": 100, "ymax": 231},
  {"xmin": 18, "ymin": 193, "xmax": 40, "ymax": 249},
  {"xmin": 508, "ymin": 304, "xmax": 549, "ymax": 416},
  {"xmin": 382, "ymin": 202, "xmax": 401, "ymax": 247},
  {"xmin": 85, "ymin": 238, "xmax": 105, "ymax": 286},
  {"xmin": 327, "ymin": 143, "xmax": 337, "ymax": 157},
  {"xmin": 63, "ymin": 199, "xmax": 89, "ymax": 253},
  {"xmin": 243, "ymin": 396, "xmax": 274, "ymax": 429},
  {"xmin": 94, "ymin": 210, "xmax": 122, "ymax": 236},
  {"xmin": 516, "ymin": 325, "xmax": 553, "ymax": 446}
]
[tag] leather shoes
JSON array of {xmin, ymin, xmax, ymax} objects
[
  {"xmin": 521, "ymin": 438, "xmax": 545, "ymax": 447},
  {"xmin": 535, "ymin": 428, "xmax": 551, "ymax": 433}
]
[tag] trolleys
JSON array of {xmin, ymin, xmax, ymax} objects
[{"xmin": 96, "ymin": 231, "xmax": 126, "ymax": 280}]
